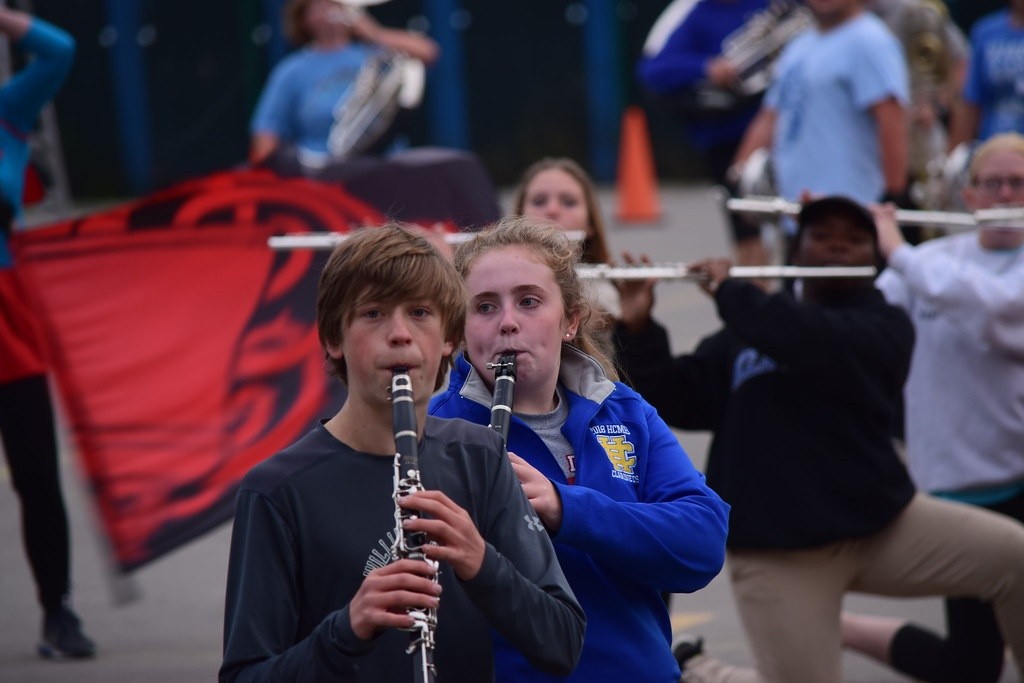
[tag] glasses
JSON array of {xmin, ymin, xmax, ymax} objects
[{"xmin": 973, "ymin": 175, "xmax": 1024, "ymax": 198}]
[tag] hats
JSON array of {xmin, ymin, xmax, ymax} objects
[{"xmin": 801, "ymin": 197, "xmax": 878, "ymax": 232}]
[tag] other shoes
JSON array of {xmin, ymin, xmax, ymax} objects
[{"xmin": 671, "ymin": 635, "xmax": 703, "ymax": 672}]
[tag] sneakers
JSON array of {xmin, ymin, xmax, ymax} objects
[{"xmin": 38, "ymin": 606, "xmax": 96, "ymax": 660}]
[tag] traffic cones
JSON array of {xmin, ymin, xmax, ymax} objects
[{"xmin": 608, "ymin": 106, "xmax": 667, "ymax": 226}]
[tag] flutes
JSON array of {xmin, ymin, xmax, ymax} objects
[
  {"xmin": 483, "ymin": 350, "xmax": 515, "ymax": 452},
  {"xmin": 728, "ymin": 196, "xmax": 1023, "ymax": 230},
  {"xmin": 267, "ymin": 230, "xmax": 591, "ymax": 250},
  {"xmin": 378, "ymin": 364, "xmax": 440, "ymax": 683},
  {"xmin": 573, "ymin": 262, "xmax": 879, "ymax": 281}
]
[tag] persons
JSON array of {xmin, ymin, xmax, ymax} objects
[
  {"xmin": 428, "ymin": 216, "xmax": 734, "ymax": 683},
  {"xmin": 0, "ymin": 2, "xmax": 99, "ymax": 663},
  {"xmin": 800, "ymin": 132, "xmax": 1024, "ymax": 683},
  {"xmin": 217, "ymin": 220, "xmax": 586, "ymax": 683},
  {"xmin": 596, "ymin": 194, "xmax": 1024, "ymax": 683},
  {"xmin": 244, "ymin": 0, "xmax": 1024, "ymax": 291}
]
